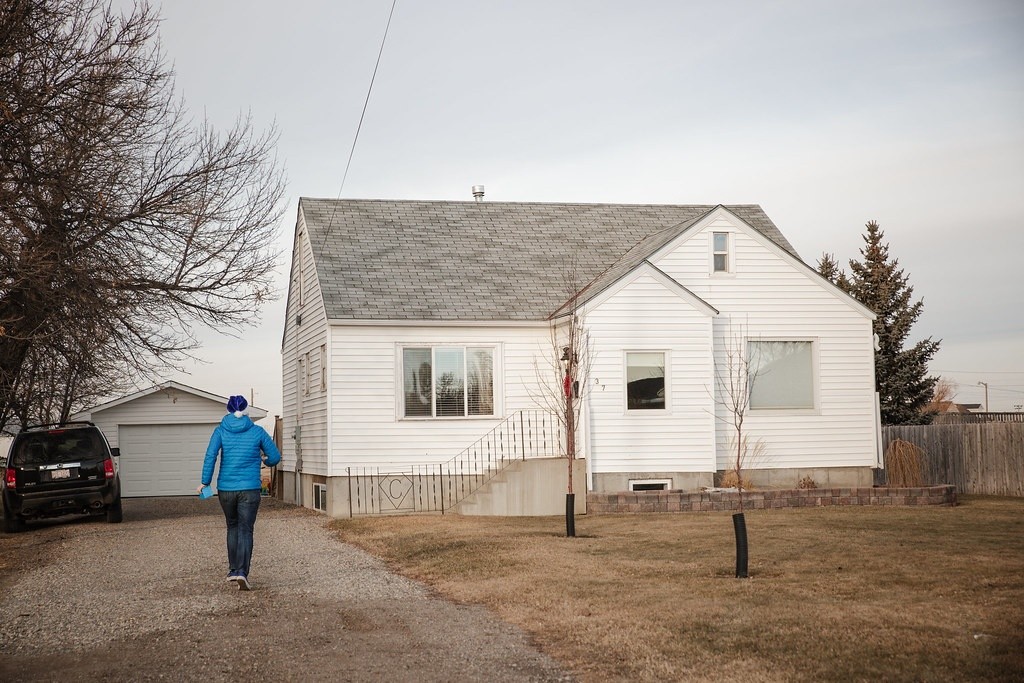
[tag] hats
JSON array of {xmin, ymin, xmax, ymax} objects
[{"xmin": 226, "ymin": 395, "xmax": 249, "ymax": 418}]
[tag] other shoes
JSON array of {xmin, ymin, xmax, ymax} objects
[
  {"xmin": 237, "ymin": 569, "xmax": 249, "ymax": 591},
  {"xmin": 226, "ymin": 571, "xmax": 238, "ymax": 581}
]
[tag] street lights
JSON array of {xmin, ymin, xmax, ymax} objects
[{"xmin": 979, "ymin": 381, "xmax": 988, "ymax": 412}]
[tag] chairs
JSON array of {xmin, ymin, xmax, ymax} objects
[{"xmin": 25, "ymin": 445, "xmax": 47, "ymax": 462}]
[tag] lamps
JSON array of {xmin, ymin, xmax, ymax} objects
[{"xmin": 560, "ymin": 347, "xmax": 578, "ymax": 374}]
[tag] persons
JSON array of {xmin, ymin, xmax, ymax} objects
[{"xmin": 197, "ymin": 395, "xmax": 280, "ymax": 591}]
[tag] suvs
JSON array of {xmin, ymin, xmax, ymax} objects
[{"xmin": 2, "ymin": 421, "xmax": 122, "ymax": 533}]
